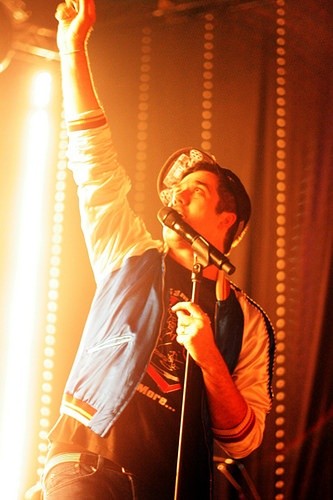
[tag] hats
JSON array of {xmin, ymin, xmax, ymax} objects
[{"xmin": 156, "ymin": 147, "xmax": 252, "ymax": 255}]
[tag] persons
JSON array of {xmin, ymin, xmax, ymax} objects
[{"xmin": 38, "ymin": 0, "xmax": 277, "ymax": 500}]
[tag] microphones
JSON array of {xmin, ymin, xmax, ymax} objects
[{"xmin": 157, "ymin": 207, "xmax": 235, "ymax": 275}]
